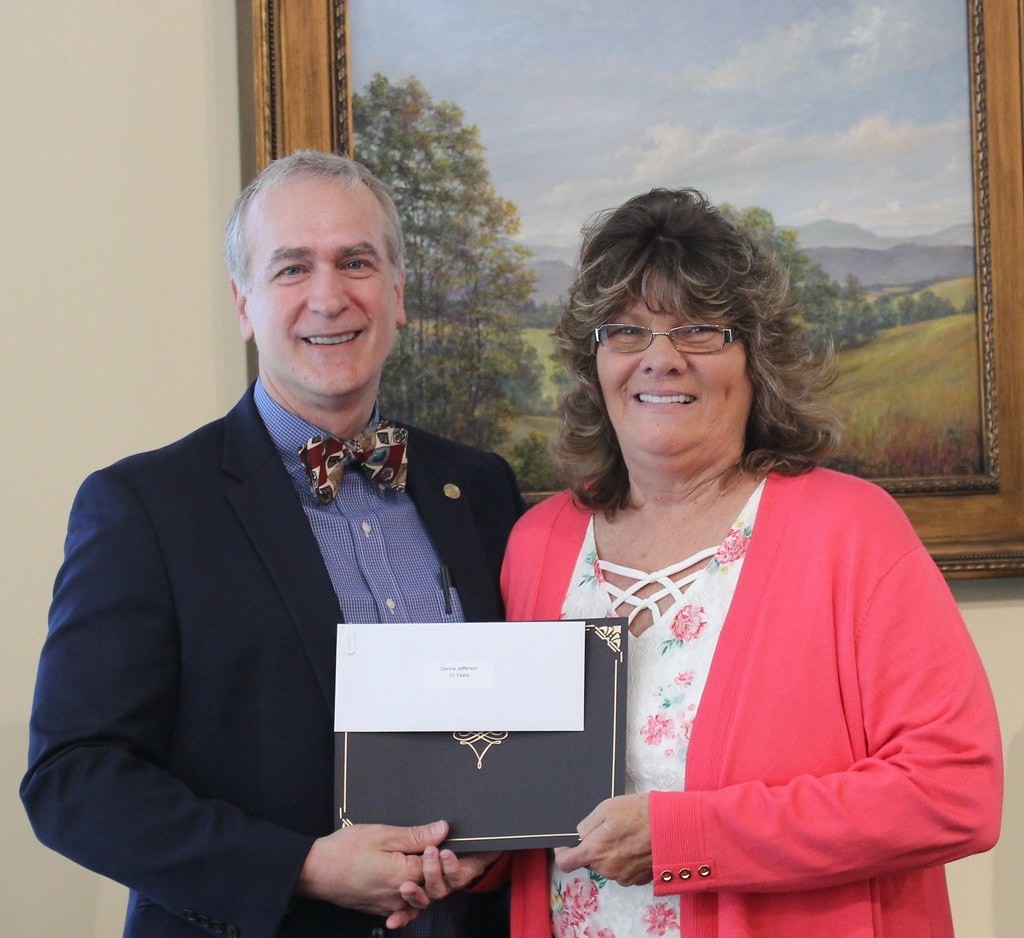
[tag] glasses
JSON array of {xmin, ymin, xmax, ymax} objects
[{"xmin": 594, "ymin": 323, "xmax": 744, "ymax": 354}]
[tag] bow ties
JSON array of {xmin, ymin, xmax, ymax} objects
[{"xmin": 296, "ymin": 419, "xmax": 408, "ymax": 506}]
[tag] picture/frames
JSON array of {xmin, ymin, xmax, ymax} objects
[{"xmin": 239, "ymin": 0, "xmax": 1024, "ymax": 581}]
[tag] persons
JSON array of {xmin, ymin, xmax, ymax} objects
[
  {"xmin": 18, "ymin": 150, "xmax": 527, "ymax": 938},
  {"xmin": 386, "ymin": 191, "xmax": 1006, "ymax": 938}
]
